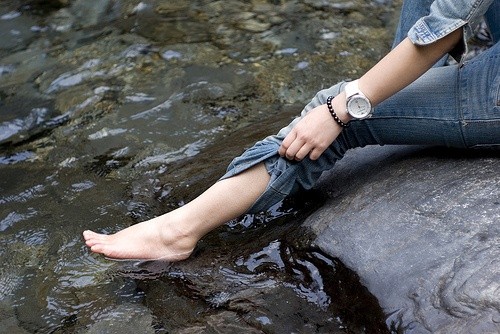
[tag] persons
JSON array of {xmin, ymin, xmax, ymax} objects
[{"xmin": 83, "ymin": 0, "xmax": 500, "ymax": 261}]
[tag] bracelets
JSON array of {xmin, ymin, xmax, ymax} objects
[{"xmin": 327, "ymin": 96, "xmax": 352, "ymax": 128}]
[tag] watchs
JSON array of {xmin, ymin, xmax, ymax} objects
[{"xmin": 345, "ymin": 80, "xmax": 374, "ymax": 121}]
